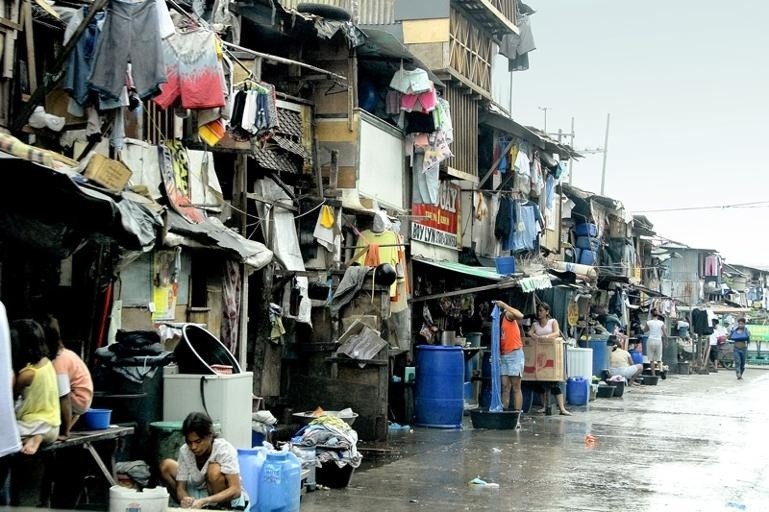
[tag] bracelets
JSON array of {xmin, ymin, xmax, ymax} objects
[{"xmin": 545, "ymin": 335, "xmax": 548, "ymax": 339}]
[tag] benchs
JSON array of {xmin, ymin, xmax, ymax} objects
[{"xmin": 41, "ymin": 427, "xmax": 134, "ymax": 484}]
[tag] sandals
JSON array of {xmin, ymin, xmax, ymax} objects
[
  {"xmin": 561, "ymin": 410, "xmax": 572, "ymax": 416},
  {"xmin": 538, "ymin": 408, "xmax": 546, "ymax": 413}
]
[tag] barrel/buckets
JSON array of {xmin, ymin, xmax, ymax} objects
[
  {"xmin": 568, "ymin": 375, "xmax": 588, "ymax": 406},
  {"xmin": 574, "ymin": 222, "xmax": 600, "ymax": 264},
  {"xmin": 236, "ymin": 446, "xmax": 267, "ymax": 512},
  {"xmin": 415, "ymin": 344, "xmax": 465, "ymax": 430},
  {"xmin": 109, "ymin": 486, "xmax": 170, "ymax": 512},
  {"xmin": 258, "ymin": 453, "xmax": 302, "ymax": 512},
  {"xmin": 630, "ymin": 348, "xmax": 643, "ymax": 364},
  {"xmin": 495, "ymin": 256, "xmax": 515, "ymax": 276},
  {"xmin": 589, "ymin": 340, "xmax": 607, "ymax": 374}
]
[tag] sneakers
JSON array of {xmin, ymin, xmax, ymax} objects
[
  {"xmin": 660, "ymin": 371, "xmax": 666, "ymax": 379},
  {"xmin": 737, "ymin": 374, "xmax": 743, "ymax": 380}
]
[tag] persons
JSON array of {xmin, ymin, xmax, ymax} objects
[
  {"xmin": 528, "ymin": 301, "xmax": 572, "ymax": 416},
  {"xmin": 727, "ymin": 317, "xmax": 750, "ymax": 380},
  {"xmin": 159, "ymin": 410, "xmax": 250, "ymax": 511},
  {"xmin": 0, "ymin": 302, "xmax": 93, "ymax": 492},
  {"xmin": 639, "ymin": 306, "xmax": 668, "ymax": 380},
  {"xmin": 495, "ymin": 300, "xmax": 525, "ymax": 430},
  {"xmin": 709, "ymin": 319, "xmax": 725, "ymax": 370},
  {"xmin": 606, "ymin": 338, "xmax": 643, "ymax": 386}
]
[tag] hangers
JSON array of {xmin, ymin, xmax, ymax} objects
[
  {"xmin": 233, "ymin": 70, "xmax": 268, "ymax": 94},
  {"xmin": 178, "ymin": 16, "xmax": 200, "ymax": 29}
]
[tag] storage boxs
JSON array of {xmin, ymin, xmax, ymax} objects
[
  {"xmin": 523, "ymin": 336, "xmax": 564, "ymax": 381},
  {"xmin": 84, "ymin": 153, "xmax": 132, "ymax": 195}
]
